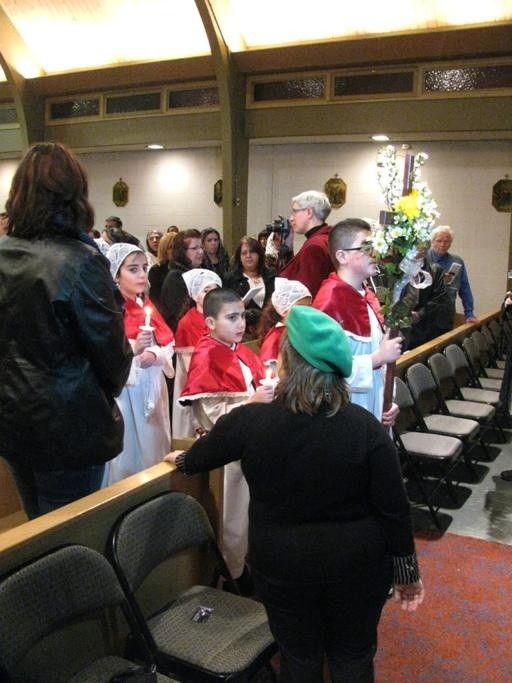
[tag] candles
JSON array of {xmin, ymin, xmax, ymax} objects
[
  {"xmin": 143, "ymin": 304, "xmax": 154, "ymax": 327},
  {"xmin": 265, "ymin": 366, "xmax": 272, "ymax": 381}
]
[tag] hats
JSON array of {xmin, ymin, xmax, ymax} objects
[
  {"xmin": 106, "ymin": 243, "xmax": 142, "ymax": 280},
  {"xmin": 181, "ymin": 269, "xmax": 222, "ymax": 301},
  {"xmin": 285, "ymin": 304, "xmax": 352, "ymax": 378},
  {"xmin": 271, "ymin": 280, "xmax": 311, "ymax": 314}
]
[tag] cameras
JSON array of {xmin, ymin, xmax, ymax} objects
[{"xmin": 266, "ymin": 213, "xmax": 290, "ymax": 233}]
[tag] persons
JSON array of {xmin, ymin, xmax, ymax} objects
[
  {"xmin": 369, "ymin": 225, "xmax": 478, "ymax": 356},
  {"xmin": 279, "ymin": 189, "xmax": 338, "ymax": 301},
  {"xmin": 93, "ymin": 218, "xmax": 313, "ymax": 581},
  {"xmin": 158, "ymin": 306, "xmax": 426, "ymax": 683},
  {"xmin": 0, "ymin": 143, "xmax": 133, "ymax": 521},
  {"xmin": 309, "ymin": 217, "xmax": 403, "ymax": 441}
]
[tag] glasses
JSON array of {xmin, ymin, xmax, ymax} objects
[{"xmin": 342, "ymin": 243, "xmax": 374, "ymax": 254}]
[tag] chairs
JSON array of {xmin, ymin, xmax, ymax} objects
[
  {"xmin": 2, "ymin": 540, "xmax": 191, "ymax": 683},
  {"xmin": 385, "ymin": 306, "xmax": 512, "ymax": 532},
  {"xmin": 105, "ymin": 488, "xmax": 282, "ymax": 683}
]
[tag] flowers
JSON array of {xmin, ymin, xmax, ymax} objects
[{"xmin": 359, "ymin": 137, "xmax": 443, "ymax": 330}]
